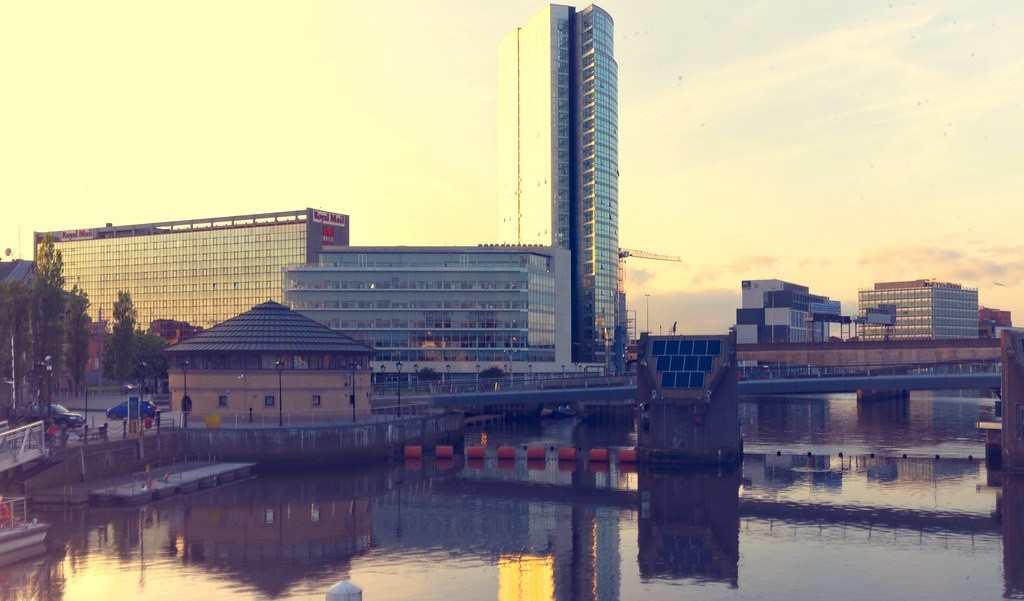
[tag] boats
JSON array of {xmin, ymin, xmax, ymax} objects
[{"xmin": 553, "ymin": 404, "xmax": 575, "ymax": 417}]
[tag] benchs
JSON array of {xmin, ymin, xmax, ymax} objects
[{"xmin": 75, "ymin": 428, "xmax": 102, "ymax": 439}]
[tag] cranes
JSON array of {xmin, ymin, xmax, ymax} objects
[{"xmin": 617, "ymin": 247, "xmax": 683, "ymax": 294}]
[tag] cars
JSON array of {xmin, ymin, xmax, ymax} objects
[
  {"xmin": 28, "ymin": 403, "xmax": 84, "ymax": 431},
  {"xmin": 118, "ymin": 384, "xmax": 139, "ymax": 395},
  {"xmin": 104, "ymin": 399, "xmax": 156, "ymax": 419}
]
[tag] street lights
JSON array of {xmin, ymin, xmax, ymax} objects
[
  {"xmin": 44, "ymin": 355, "xmax": 55, "ymax": 452},
  {"xmin": 476, "ymin": 364, "xmax": 480, "ymax": 385},
  {"xmin": 504, "ymin": 364, "xmax": 508, "ymax": 380},
  {"xmin": 578, "ymin": 364, "xmax": 583, "ymax": 376},
  {"xmin": 380, "ymin": 364, "xmax": 385, "ymax": 396},
  {"xmin": 645, "ymin": 294, "xmax": 651, "ymax": 333},
  {"xmin": 275, "ymin": 360, "xmax": 284, "ymax": 428},
  {"xmin": 446, "ymin": 364, "xmax": 450, "ymax": 382},
  {"xmin": 181, "ymin": 361, "xmax": 192, "ymax": 430},
  {"xmin": 349, "ymin": 361, "xmax": 358, "ymax": 423},
  {"xmin": 138, "ymin": 362, "xmax": 149, "ymax": 436},
  {"xmin": 413, "ymin": 364, "xmax": 419, "ymax": 392},
  {"xmin": 562, "ymin": 365, "xmax": 565, "ymax": 383},
  {"xmin": 395, "ymin": 360, "xmax": 403, "ymax": 417},
  {"xmin": 528, "ymin": 364, "xmax": 533, "ymax": 383}
]
